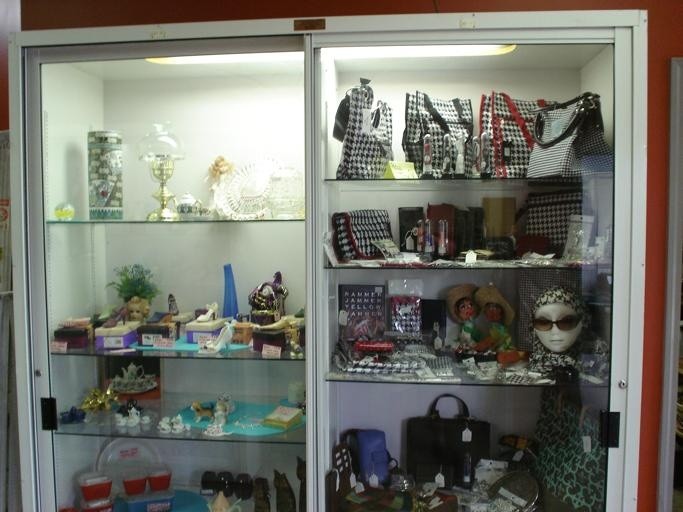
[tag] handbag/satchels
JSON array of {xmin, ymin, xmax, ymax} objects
[
  {"xmin": 405, "ymin": 394, "xmax": 490, "ymax": 487},
  {"xmin": 459, "ymin": 249, "xmax": 496, "ymax": 260},
  {"xmin": 526, "ymin": 92, "xmax": 614, "ymax": 178},
  {"xmin": 533, "ymin": 386, "xmax": 605, "ymax": 511},
  {"xmin": 340, "ymin": 429, "xmax": 391, "ymax": 486},
  {"xmin": 401, "ymin": 97, "xmax": 474, "ymax": 174},
  {"xmin": 356, "ymin": 341, "xmax": 395, "ymax": 352},
  {"xmin": 333, "ymin": 206, "xmax": 395, "ymax": 261},
  {"xmin": 417, "ymin": 491, "xmax": 458, "ymax": 511},
  {"xmin": 526, "ymin": 188, "xmax": 589, "ymax": 247},
  {"xmin": 334, "ymin": 76, "xmax": 396, "ymax": 178},
  {"xmin": 479, "ymin": 92, "xmax": 561, "ymax": 176}
]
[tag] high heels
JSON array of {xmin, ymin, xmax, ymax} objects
[
  {"xmin": 103, "ymin": 306, "xmax": 127, "ymax": 328},
  {"xmin": 197, "ymin": 301, "xmax": 218, "ymax": 322},
  {"xmin": 198, "ymin": 320, "xmax": 236, "ymax": 354}
]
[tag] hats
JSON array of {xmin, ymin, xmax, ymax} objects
[
  {"xmin": 446, "ymin": 284, "xmax": 472, "ymax": 323},
  {"xmin": 475, "ymin": 284, "xmax": 515, "ymax": 326}
]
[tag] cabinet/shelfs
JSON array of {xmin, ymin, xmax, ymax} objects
[
  {"xmin": 20, "ymin": 22, "xmax": 317, "ymax": 512},
  {"xmin": 315, "ymin": 6, "xmax": 645, "ymax": 512}
]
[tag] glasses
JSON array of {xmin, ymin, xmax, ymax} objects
[{"xmin": 530, "ymin": 314, "xmax": 583, "ymax": 331}]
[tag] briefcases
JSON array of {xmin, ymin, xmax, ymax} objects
[
  {"xmin": 469, "ymin": 206, "xmax": 484, "ymax": 250},
  {"xmin": 427, "ymin": 203, "xmax": 454, "ymax": 257},
  {"xmin": 482, "ymin": 196, "xmax": 516, "ymax": 239},
  {"xmin": 455, "ymin": 210, "xmax": 474, "ymax": 251},
  {"xmin": 398, "ymin": 205, "xmax": 423, "ymax": 251}
]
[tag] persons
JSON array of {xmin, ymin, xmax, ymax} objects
[
  {"xmin": 127, "ymin": 297, "xmax": 150, "ymax": 322},
  {"xmin": 532, "ymin": 285, "xmax": 588, "ymax": 353},
  {"xmin": 483, "ymin": 301, "xmax": 512, "ymax": 353},
  {"xmin": 451, "ymin": 294, "xmax": 480, "ymax": 352}
]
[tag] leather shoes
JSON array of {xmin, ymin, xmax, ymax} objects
[
  {"xmin": 168, "ymin": 293, "xmax": 179, "ymax": 316},
  {"xmin": 260, "ymin": 314, "xmax": 296, "ymax": 332}
]
